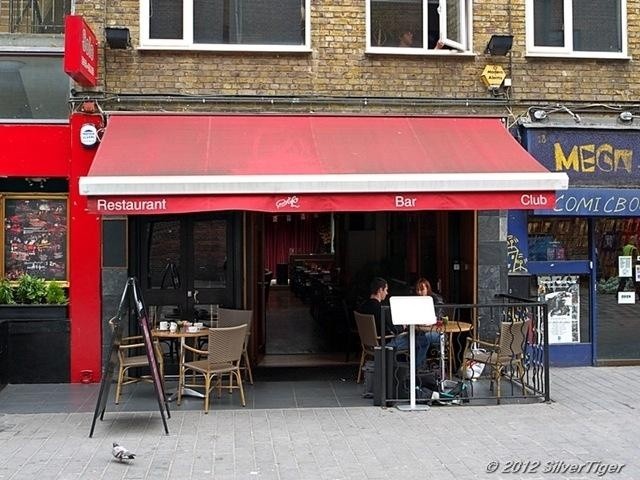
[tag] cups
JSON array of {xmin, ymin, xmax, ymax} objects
[
  {"xmin": 160, "ymin": 321, "xmax": 170, "ymax": 330},
  {"xmin": 187, "ymin": 327, "xmax": 200, "ymax": 332},
  {"xmin": 305, "ymin": 262, "xmax": 322, "ymax": 273},
  {"xmin": 194, "ymin": 323, "xmax": 204, "ymax": 328},
  {"xmin": 289, "ymin": 248, "xmax": 294, "ymax": 254}
]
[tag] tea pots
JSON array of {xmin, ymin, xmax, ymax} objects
[{"xmin": 170, "ymin": 321, "xmax": 177, "ymax": 333}]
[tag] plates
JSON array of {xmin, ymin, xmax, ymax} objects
[
  {"xmin": 159, "ymin": 329, "xmax": 169, "ymax": 332},
  {"xmin": 198, "ymin": 327, "xmax": 208, "ymax": 330},
  {"xmin": 187, "ymin": 332, "xmax": 200, "ymax": 334}
]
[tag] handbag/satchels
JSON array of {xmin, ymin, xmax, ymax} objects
[
  {"xmin": 439, "ymin": 379, "xmax": 469, "ymax": 403},
  {"xmin": 457, "ymin": 342, "xmax": 488, "ymax": 381}
]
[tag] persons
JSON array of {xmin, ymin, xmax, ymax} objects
[
  {"xmin": 359, "ymin": 277, "xmax": 430, "ymax": 372},
  {"xmin": 396, "ymin": 29, "xmax": 442, "ymax": 49},
  {"xmin": 414, "ymin": 278, "xmax": 445, "ymax": 355},
  {"xmin": 615, "ymin": 236, "xmax": 640, "ymax": 301}
]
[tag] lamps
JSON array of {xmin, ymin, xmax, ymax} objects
[
  {"xmin": 104, "ymin": 26, "xmax": 132, "ymax": 50},
  {"xmin": 485, "ymin": 33, "xmax": 515, "ymax": 56}
]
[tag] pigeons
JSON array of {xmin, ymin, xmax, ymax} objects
[{"xmin": 112, "ymin": 442, "xmax": 136, "ymax": 463}]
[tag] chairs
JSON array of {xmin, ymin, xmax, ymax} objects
[
  {"xmin": 353, "ymin": 312, "xmax": 411, "ymax": 384},
  {"xmin": 462, "ymin": 319, "xmax": 532, "ymax": 405},
  {"xmin": 108, "ymin": 315, "xmax": 164, "ymax": 405},
  {"xmin": 179, "ymin": 324, "xmax": 248, "ymax": 414},
  {"xmin": 615, "ymin": 245, "xmax": 640, "ymax": 301},
  {"xmin": 194, "ymin": 308, "xmax": 256, "ymax": 385}
]
